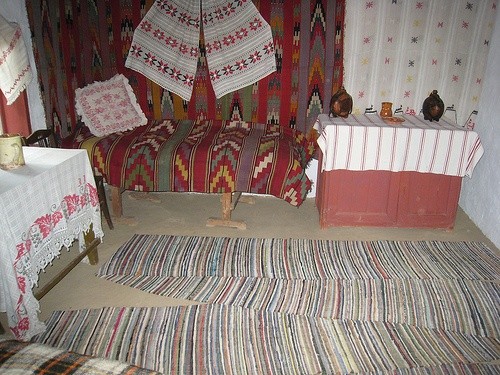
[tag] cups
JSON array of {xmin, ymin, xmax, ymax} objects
[{"xmin": 0, "ymin": 133, "xmax": 25, "ymax": 170}]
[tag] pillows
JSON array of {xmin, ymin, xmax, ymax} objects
[{"xmin": 75, "ymin": 74, "xmax": 148, "ymax": 137}]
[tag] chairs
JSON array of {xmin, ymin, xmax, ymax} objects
[{"xmin": 20, "ymin": 124, "xmax": 114, "ymax": 230}]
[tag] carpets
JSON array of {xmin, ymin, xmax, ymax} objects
[{"xmin": 27, "ymin": 233, "xmax": 500, "ymax": 375}]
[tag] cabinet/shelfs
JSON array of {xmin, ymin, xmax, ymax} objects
[{"xmin": 313, "ymin": 114, "xmax": 485, "ymax": 233}]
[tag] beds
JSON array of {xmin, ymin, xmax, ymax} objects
[{"xmin": 62, "ymin": 118, "xmax": 314, "ymax": 231}]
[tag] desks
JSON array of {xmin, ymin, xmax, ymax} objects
[{"xmin": 0, "ymin": 146, "xmax": 105, "ymax": 342}]
